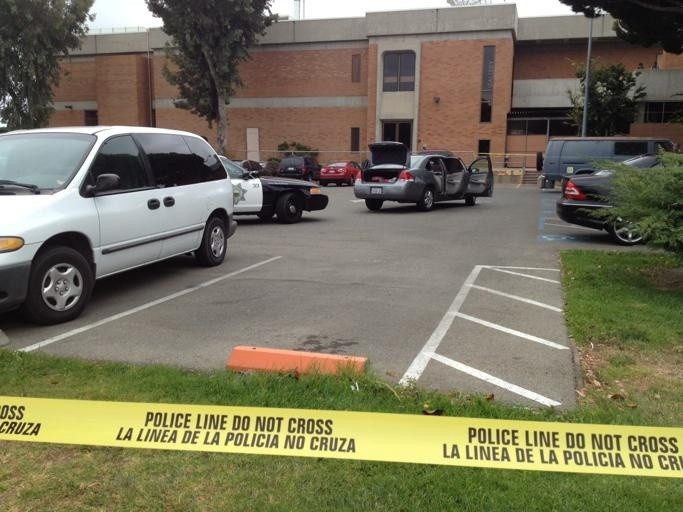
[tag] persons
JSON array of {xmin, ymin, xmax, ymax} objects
[{"xmin": 637, "ymin": 61, "xmax": 659, "ymax": 69}]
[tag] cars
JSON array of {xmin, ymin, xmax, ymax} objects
[
  {"xmin": 556, "ymin": 153, "xmax": 676, "ymax": 245},
  {"xmin": 220, "ymin": 140, "xmax": 494, "ymax": 223}
]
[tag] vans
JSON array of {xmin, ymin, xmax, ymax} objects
[
  {"xmin": 0, "ymin": 124, "xmax": 239, "ymax": 326},
  {"xmin": 535, "ymin": 136, "xmax": 681, "ymax": 189}
]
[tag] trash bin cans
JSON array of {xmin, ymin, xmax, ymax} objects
[{"xmin": 544, "ymin": 178, "xmax": 554, "ymax": 188}]
